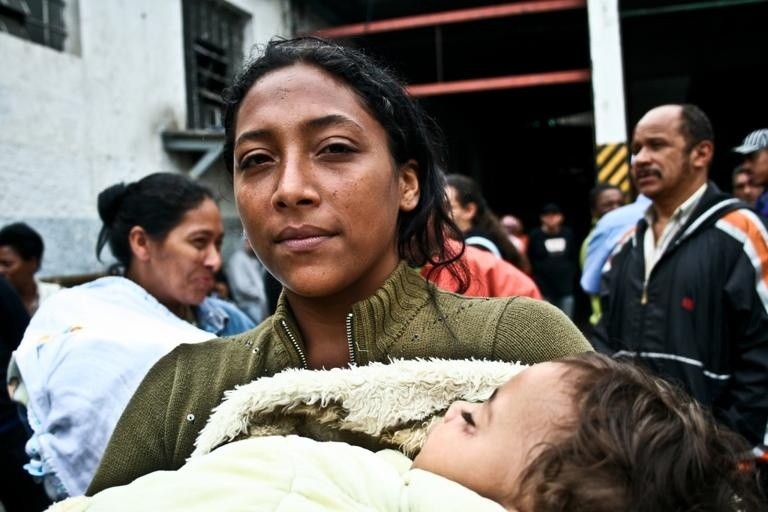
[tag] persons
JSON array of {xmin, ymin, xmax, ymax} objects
[
  {"xmin": 41, "ymin": 347, "xmax": 758, "ymax": 512},
  {"xmin": 10, "ymin": 169, "xmax": 258, "ymax": 499},
  {"xmin": 589, "ymin": 102, "xmax": 767, "ymax": 512},
  {"xmin": 81, "ymin": 30, "xmax": 599, "ymax": 502},
  {"xmin": 0, "ymin": 271, "xmax": 55, "ymax": 512}
]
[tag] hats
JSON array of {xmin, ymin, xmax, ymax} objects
[{"xmin": 732, "ymin": 128, "xmax": 768, "ymax": 155}]
